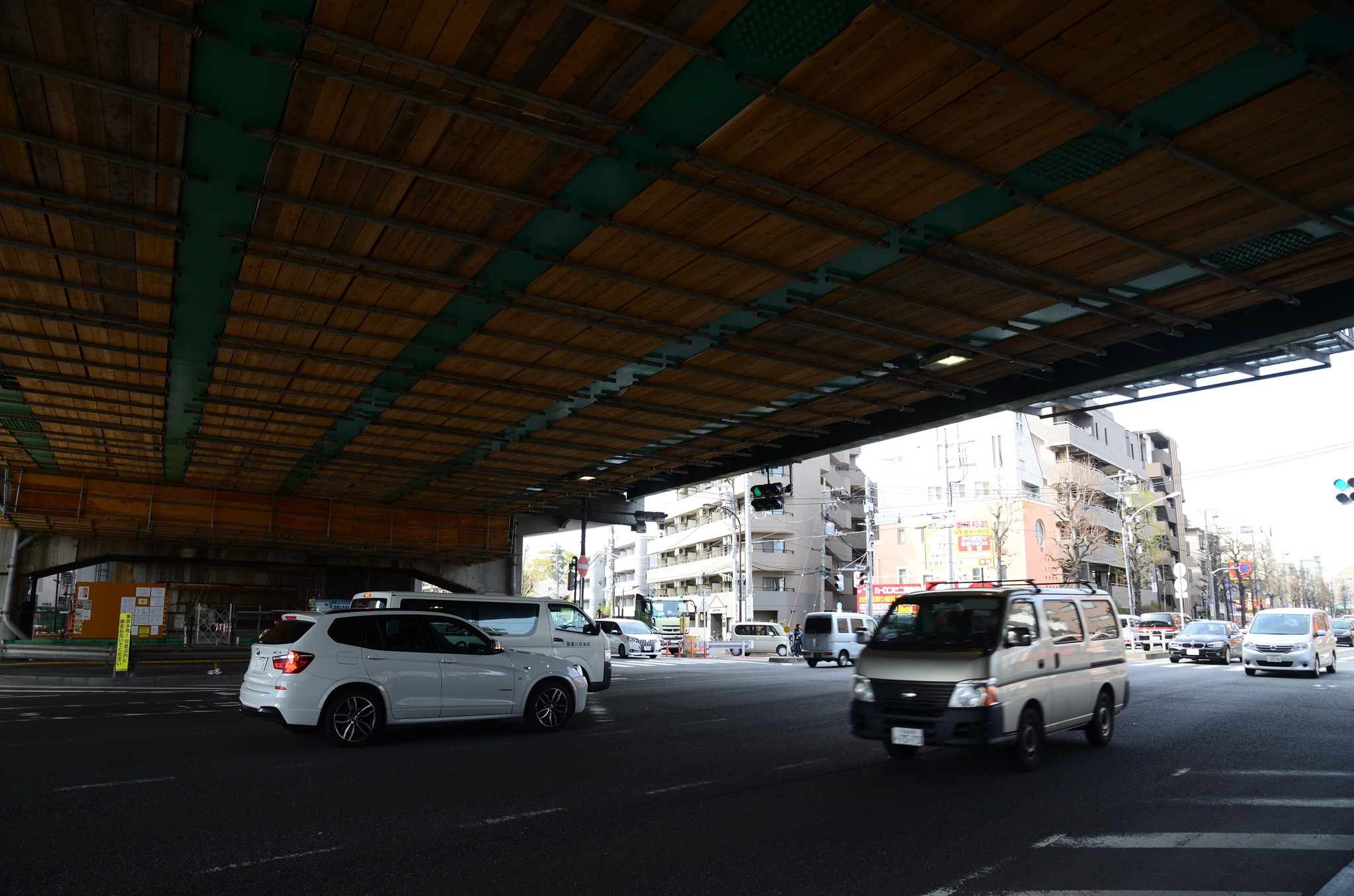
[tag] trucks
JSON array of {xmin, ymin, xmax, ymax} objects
[{"xmin": 612, "ymin": 594, "xmax": 699, "ymax": 655}]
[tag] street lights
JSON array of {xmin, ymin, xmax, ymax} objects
[
  {"xmin": 1332, "ymin": 581, "xmax": 1353, "ymax": 619},
  {"xmin": 1301, "ymin": 569, "xmax": 1328, "ymax": 609},
  {"xmin": 1253, "ymin": 553, "xmax": 1290, "ymax": 612},
  {"xmin": 53, "ymin": 579, "xmax": 77, "ymax": 638},
  {"xmin": 703, "ymin": 503, "xmax": 741, "ymax": 623},
  {"xmin": 1122, "ymin": 492, "xmax": 1182, "ymax": 616},
  {"xmin": 621, "ymin": 585, "xmax": 639, "ymax": 618}
]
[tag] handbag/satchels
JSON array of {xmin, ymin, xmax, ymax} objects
[{"xmin": 795, "ymin": 640, "xmax": 801, "ymax": 644}]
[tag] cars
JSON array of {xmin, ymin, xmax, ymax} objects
[
  {"xmin": 239, "ymin": 606, "xmax": 588, "ymax": 748},
  {"xmin": 593, "ymin": 618, "xmax": 662, "ymax": 659},
  {"xmin": 1168, "ymin": 620, "xmax": 1244, "ymax": 665},
  {"xmin": 1329, "ymin": 615, "xmax": 1354, "ymax": 647}
]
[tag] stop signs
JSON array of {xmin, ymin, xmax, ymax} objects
[{"xmin": 577, "ymin": 555, "xmax": 588, "ymax": 578}]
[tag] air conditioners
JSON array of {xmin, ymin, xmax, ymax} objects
[
  {"xmin": 657, "ymin": 553, "xmax": 667, "ymax": 560},
  {"xmin": 696, "ymin": 577, "xmax": 709, "ymax": 585},
  {"xmin": 674, "ymin": 516, "xmax": 687, "ymax": 525},
  {"xmin": 655, "ymin": 583, "xmax": 666, "ymax": 590},
  {"xmin": 674, "ymin": 580, "xmax": 686, "ymax": 587},
  {"xmin": 696, "ymin": 542, "xmax": 709, "ymax": 551},
  {"xmin": 697, "ymin": 508, "xmax": 709, "ymax": 518},
  {"xmin": 674, "ymin": 548, "xmax": 685, "ymax": 556}
]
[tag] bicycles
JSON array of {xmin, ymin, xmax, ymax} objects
[{"xmin": 709, "ymin": 631, "xmax": 723, "ymax": 641}]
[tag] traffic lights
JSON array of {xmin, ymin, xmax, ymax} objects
[
  {"xmin": 834, "ymin": 574, "xmax": 844, "ymax": 591},
  {"xmin": 854, "ymin": 572, "xmax": 865, "ymax": 588},
  {"xmin": 751, "ymin": 496, "xmax": 784, "ymax": 512},
  {"xmin": 1334, "ymin": 478, "xmax": 1354, "ymax": 504},
  {"xmin": 750, "ymin": 482, "xmax": 783, "ymax": 498}
]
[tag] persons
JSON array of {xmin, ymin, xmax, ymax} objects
[
  {"xmin": 740, "ymin": 627, "xmax": 765, "ymax": 636},
  {"xmin": 788, "ymin": 623, "xmax": 804, "ymax": 657},
  {"xmin": 918, "ymin": 608, "xmax": 956, "ymax": 644}
]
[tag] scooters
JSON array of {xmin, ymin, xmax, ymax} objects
[{"xmin": 788, "ymin": 632, "xmax": 804, "ymax": 657}]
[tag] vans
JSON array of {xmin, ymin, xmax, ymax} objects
[
  {"xmin": 802, "ymin": 612, "xmax": 878, "ymax": 667},
  {"xmin": 1117, "ymin": 612, "xmax": 1194, "ymax": 651},
  {"xmin": 1241, "ymin": 608, "xmax": 1337, "ymax": 678},
  {"xmin": 730, "ymin": 622, "xmax": 790, "ymax": 656},
  {"xmin": 849, "ymin": 579, "xmax": 1130, "ymax": 770},
  {"xmin": 349, "ymin": 591, "xmax": 622, "ymax": 692}
]
